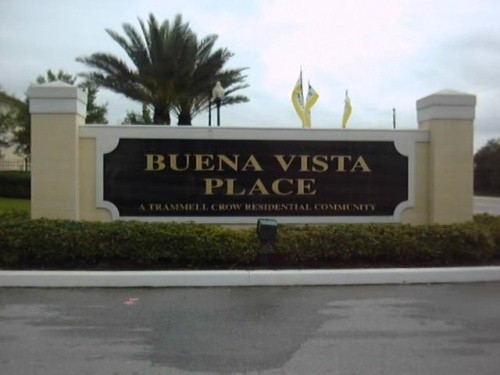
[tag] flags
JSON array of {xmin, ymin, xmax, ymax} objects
[
  {"xmin": 305, "ymin": 83, "xmax": 320, "ymax": 128},
  {"xmin": 342, "ymin": 92, "xmax": 352, "ymax": 129},
  {"xmin": 291, "ymin": 74, "xmax": 307, "ymax": 128}
]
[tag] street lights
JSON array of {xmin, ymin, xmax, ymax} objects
[{"xmin": 212, "ymin": 80, "xmax": 224, "ymax": 128}]
